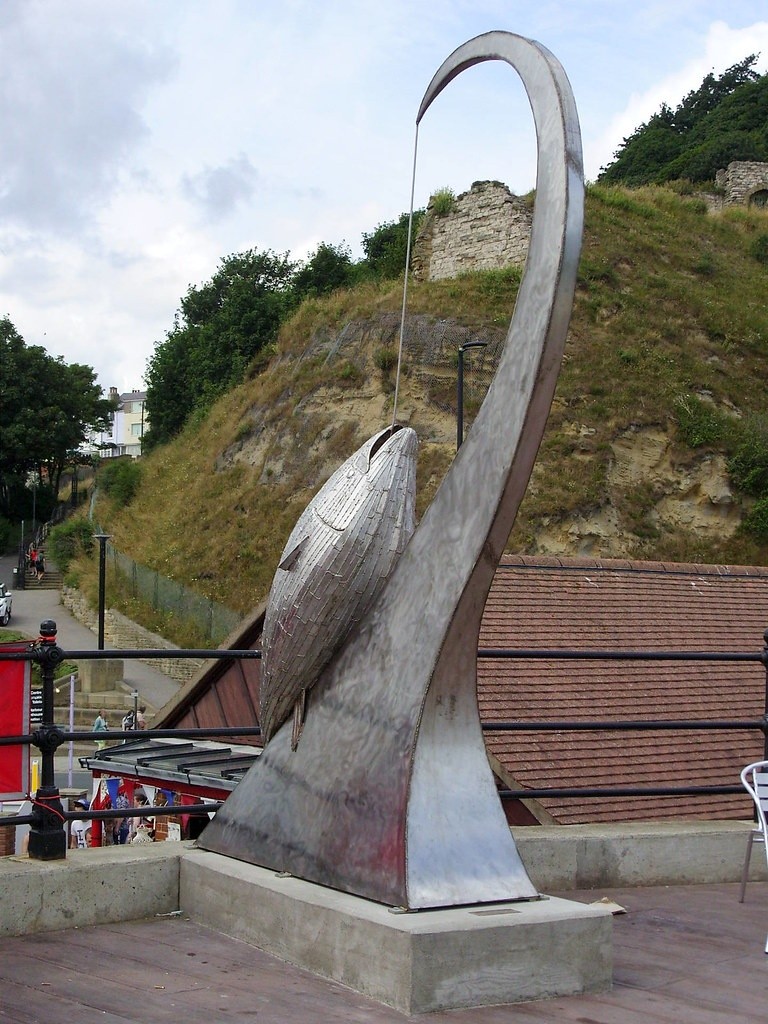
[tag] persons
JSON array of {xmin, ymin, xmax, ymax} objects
[
  {"xmin": 70, "ymin": 793, "xmax": 156, "ymax": 849},
  {"xmin": 92, "ymin": 709, "xmax": 109, "ymax": 751},
  {"xmin": 25, "ymin": 548, "xmax": 46, "ymax": 584},
  {"xmin": 121, "ymin": 705, "xmax": 146, "ymax": 730}
]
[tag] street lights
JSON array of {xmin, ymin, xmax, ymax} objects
[
  {"xmin": 140, "ymin": 399, "xmax": 147, "ymax": 455},
  {"xmin": 455, "ymin": 341, "xmax": 491, "ymax": 453},
  {"xmin": 90, "ymin": 533, "xmax": 113, "ymax": 651},
  {"xmin": 28, "ymin": 458, "xmax": 40, "ymax": 532}
]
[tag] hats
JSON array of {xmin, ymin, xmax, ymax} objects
[{"xmin": 73, "ymin": 799, "xmax": 90, "ymax": 809}]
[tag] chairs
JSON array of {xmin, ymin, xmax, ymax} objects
[{"xmin": 736, "ymin": 760, "xmax": 768, "ymax": 904}]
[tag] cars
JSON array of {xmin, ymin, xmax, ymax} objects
[{"xmin": 0, "ymin": 581, "xmax": 14, "ymax": 627}]
